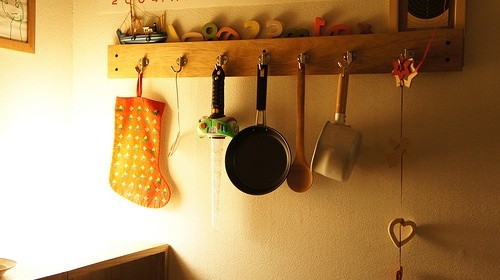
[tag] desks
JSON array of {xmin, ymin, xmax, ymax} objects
[{"xmin": 0, "ymin": 209, "xmax": 171, "ymax": 280}]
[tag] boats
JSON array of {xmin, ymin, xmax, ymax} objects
[{"xmin": 115, "ymin": 0, "xmax": 167, "ymax": 43}]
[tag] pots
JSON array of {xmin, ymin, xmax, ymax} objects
[{"xmin": 224, "ymin": 64, "xmax": 292, "ymax": 196}]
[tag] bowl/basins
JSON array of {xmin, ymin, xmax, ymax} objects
[{"xmin": 0, "ymin": 258, "xmax": 16, "ymax": 279}]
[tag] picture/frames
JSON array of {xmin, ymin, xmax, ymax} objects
[{"xmin": 0, "ymin": 0, "xmax": 36, "ymax": 54}]
